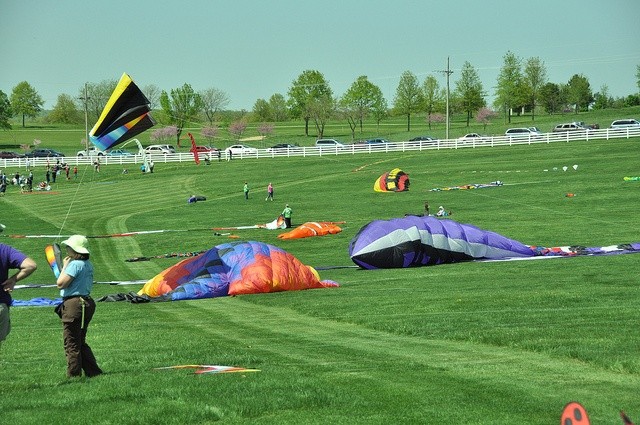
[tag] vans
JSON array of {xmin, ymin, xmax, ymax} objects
[
  {"xmin": 609, "ymin": 119, "xmax": 640, "ymax": 133},
  {"xmin": 553, "ymin": 124, "xmax": 590, "ymax": 135},
  {"xmin": 315, "ymin": 139, "xmax": 345, "ymax": 150},
  {"xmin": 505, "ymin": 128, "xmax": 540, "ymax": 140}
]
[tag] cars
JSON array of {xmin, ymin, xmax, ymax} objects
[
  {"xmin": 409, "ymin": 136, "xmax": 443, "ymax": 146},
  {"xmin": 459, "ymin": 133, "xmax": 491, "ymax": 144},
  {"xmin": 77, "ymin": 147, "xmax": 109, "ymax": 156},
  {"xmin": 162, "ymin": 145, "xmax": 175, "ymax": 153},
  {"xmin": 366, "ymin": 138, "xmax": 397, "ymax": 148},
  {"xmin": 28, "ymin": 149, "xmax": 65, "ymax": 157},
  {"xmin": 530, "ymin": 127, "xmax": 540, "ymax": 134},
  {"xmin": 269, "ymin": 144, "xmax": 301, "ymax": 152},
  {"xmin": 225, "ymin": 145, "xmax": 258, "ymax": 154},
  {"xmin": 0, "ymin": 151, "xmax": 25, "ymax": 159},
  {"xmin": 106, "ymin": 149, "xmax": 135, "ymax": 157},
  {"xmin": 138, "ymin": 145, "xmax": 171, "ymax": 155},
  {"xmin": 190, "ymin": 146, "xmax": 222, "ymax": 155}
]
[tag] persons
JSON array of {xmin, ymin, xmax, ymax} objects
[
  {"xmin": 3, "ymin": 172, "xmax": 6, "ymax": 184},
  {"xmin": 425, "ymin": 201, "xmax": 431, "ymax": 216},
  {"xmin": 281, "ymin": 204, "xmax": 293, "ymax": 229},
  {"xmin": 74, "ymin": 167, "xmax": 78, "ymax": 179},
  {"xmin": 96, "ymin": 158, "xmax": 101, "ymax": 172},
  {"xmin": 29, "ymin": 172, "xmax": 33, "ymax": 180},
  {"xmin": 56, "ymin": 234, "xmax": 103, "ymax": 377},
  {"xmin": 218, "ymin": 151, "xmax": 221, "ymax": 161},
  {"xmin": 46, "ymin": 170, "xmax": 50, "ymax": 185},
  {"xmin": 51, "ymin": 169, "xmax": 56, "ymax": 183},
  {"xmin": 263, "ymin": 183, "xmax": 274, "ymax": 201},
  {"xmin": 27, "ymin": 159, "xmax": 31, "ymax": 171},
  {"xmin": 229, "ymin": 149, "xmax": 233, "ymax": 160},
  {"xmin": 65, "ymin": 166, "xmax": 70, "ymax": 178},
  {"xmin": 139, "ymin": 163, "xmax": 147, "ymax": 173},
  {"xmin": 244, "ymin": 180, "xmax": 249, "ymax": 200},
  {"xmin": 15, "ymin": 172, "xmax": 20, "ymax": 185},
  {"xmin": 149, "ymin": 159, "xmax": 155, "ymax": 172},
  {"xmin": 0, "ymin": 223, "xmax": 38, "ymax": 345},
  {"xmin": 434, "ymin": 206, "xmax": 444, "ymax": 216},
  {"xmin": 56, "ymin": 158, "xmax": 59, "ymax": 164},
  {"xmin": 46, "ymin": 161, "xmax": 50, "ymax": 170},
  {"xmin": 59, "ymin": 163, "xmax": 64, "ymax": 170},
  {"xmin": 27, "ymin": 178, "xmax": 32, "ymax": 189}
]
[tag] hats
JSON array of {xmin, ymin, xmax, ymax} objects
[{"xmin": 61, "ymin": 235, "xmax": 90, "ymax": 254}]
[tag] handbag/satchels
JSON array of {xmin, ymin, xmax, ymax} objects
[{"xmin": 54, "ymin": 301, "xmax": 64, "ymax": 318}]
